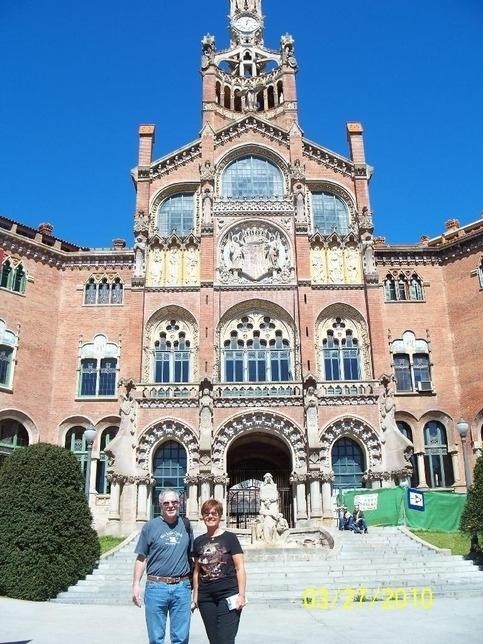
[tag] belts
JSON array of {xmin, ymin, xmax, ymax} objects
[{"xmin": 147, "ymin": 575, "xmax": 188, "ymax": 583}]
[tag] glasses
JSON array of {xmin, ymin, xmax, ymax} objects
[
  {"xmin": 160, "ymin": 500, "xmax": 179, "ymax": 506},
  {"xmin": 205, "ymin": 512, "xmax": 217, "ymax": 516}
]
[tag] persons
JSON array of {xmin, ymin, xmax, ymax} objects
[
  {"xmin": 264, "ymin": 236, "xmax": 279, "ymax": 265},
  {"xmin": 133, "ymin": 236, "xmax": 145, "ymax": 277},
  {"xmin": 312, "ymin": 246, "xmax": 358, "ymax": 282},
  {"xmin": 199, "ymin": 388, "xmax": 213, "ymax": 451},
  {"xmin": 296, "ymin": 183, "xmax": 306, "ymax": 222},
  {"xmin": 151, "ymin": 247, "xmax": 198, "ymax": 285},
  {"xmin": 339, "ymin": 505, "xmax": 368, "ymax": 534},
  {"xmin": 362, "ymin": 235, "xmax": 377, "ymax": 274},
  {"xmin": 202, "ymin": 188, "xmax": 213, "ymax": 223},
  {"xmin": 133, "ymin": 488, "xmax": 196, "ymax": 644},
  {"xmin": 230, "ymin": 235, "xmax": 243, "ymax": 265},
  {"xmin": 256, "ymin": 473, "xmax": 290, "ymax": 543},
  {"xmin": 104, "ymin": 392, "xmax": 131, "ymax": 465},
  {"xmin": 193, "ymin": 500, "xmax": 246, "ymax": 644},
  {"xmin": 304, "ymin": 386, "xmax": 321, "ymax": 448},
  {"xmin": 384, "ymin": 388, "xmax": 414, "ymax": 460}
]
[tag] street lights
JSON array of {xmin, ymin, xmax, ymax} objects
[
  {"xmin": 456, "ymin": 417, "xmax": 474, "ymax": 491},
  {"xmin": 84, "ymin": 422, "xmax": 97, "ymax": 498}
]
